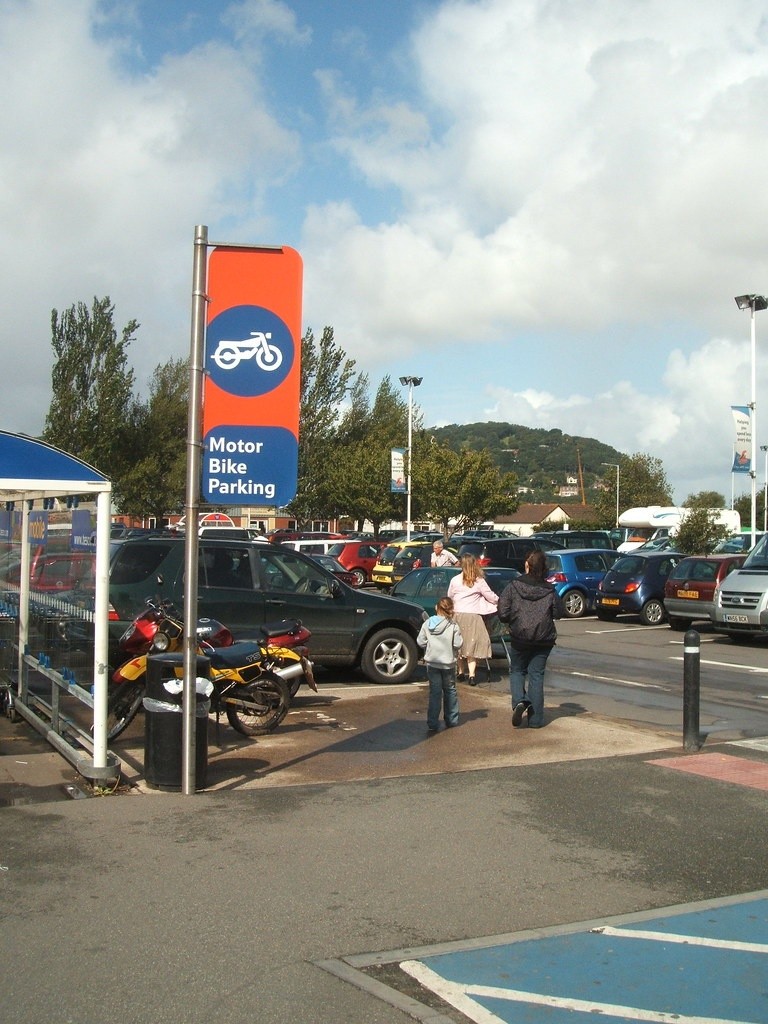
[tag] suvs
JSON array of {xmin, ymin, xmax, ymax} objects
[{"xmin": 65, "ymin": 534, "xmax": 428, "ymax": 686}]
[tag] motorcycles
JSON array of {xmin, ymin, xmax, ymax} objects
[{"xmin": 79, "ymin": 570, "xmax": 319, "ymax": 743}]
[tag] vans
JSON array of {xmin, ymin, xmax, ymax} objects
[
  {"xmin": 528, "ymin": 530, "xmax": 615, "ymax": 570},
  {"xmin": 614, "ymin": 504, "xmax": 742, "ymax": 556},
  {"xmin": 458, "ymin": 538, "xmax": 572, "ymax": 574}
]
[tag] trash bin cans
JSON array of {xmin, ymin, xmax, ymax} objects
[{"xmin": 144, "ymin": 653, "xmax": 212, "ymax": 792}]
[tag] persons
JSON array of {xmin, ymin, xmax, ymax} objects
[
  {"xmin": 447, "ymin": 552, "xmax": 499, "ymax": 685},
  {"xmin": 497, "ymin": 549, "xmax": 561, "ymax": 729},
  {"xmin": 417, "ymin": 597, "xmax": 464, "ymax": 731},
  {"xmin": 252, "ymin": 530, "xmax": 270, "ymax": 544},
  {"xmin": 431, "ymin": 541, "xmax": 461, "ymax": 567}
]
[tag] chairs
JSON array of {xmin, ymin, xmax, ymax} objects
[{"xmin": 209, "ymin": 555, "xmax": 270, "ymax": 588}]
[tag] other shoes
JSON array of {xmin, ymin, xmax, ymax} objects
[
  {"xmin": 457, "ymin": 672, "xmax": 466, "ymax": 682},
  {"xmin": 512, "ymin": 703, "xmax": 526, "ymax": 726},
  {"xmin": 469, "ymin": 677, "xmax": 477, "ymax": 686}
]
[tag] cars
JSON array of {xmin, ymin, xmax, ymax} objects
[
  {"xmin": 713, "ymin": 531, "xmax": 768, "ymax": 645},
  {"xmin": 387, "ymin": 565, "xmax": 520, "ymax": 646},
  {"xmin": 662, "ymin": 553, "xmax": 751, "ymax": 633},
  {"xmin": 543, "ymin": 549, "xmax": 629, "ymax": 619},
  {"xmin": 1, "ymin": 522, "xmax": 520, "ymax": 589},
  {"xmin": 594, "ymin": 554, "xmax": 686, "ymax": 626},
  {"xmin": 628, "ymin": 530, "xmax": 768, "ymax": 555},
  {"xmin": 609, "ymin": 527, "xmax": 636, "ymax": 549}
]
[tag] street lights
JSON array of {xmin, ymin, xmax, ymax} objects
[
  {"xmin": 399, "ymin": 374, "xmax": 424, "ymax": 543},
  {"xmin": 733, "ymin": 293, "xmax": 768, "ymax": 552},
  {"xmin": 598, "ymin": 462, "xmax": 620, "ymax": 526}
]
